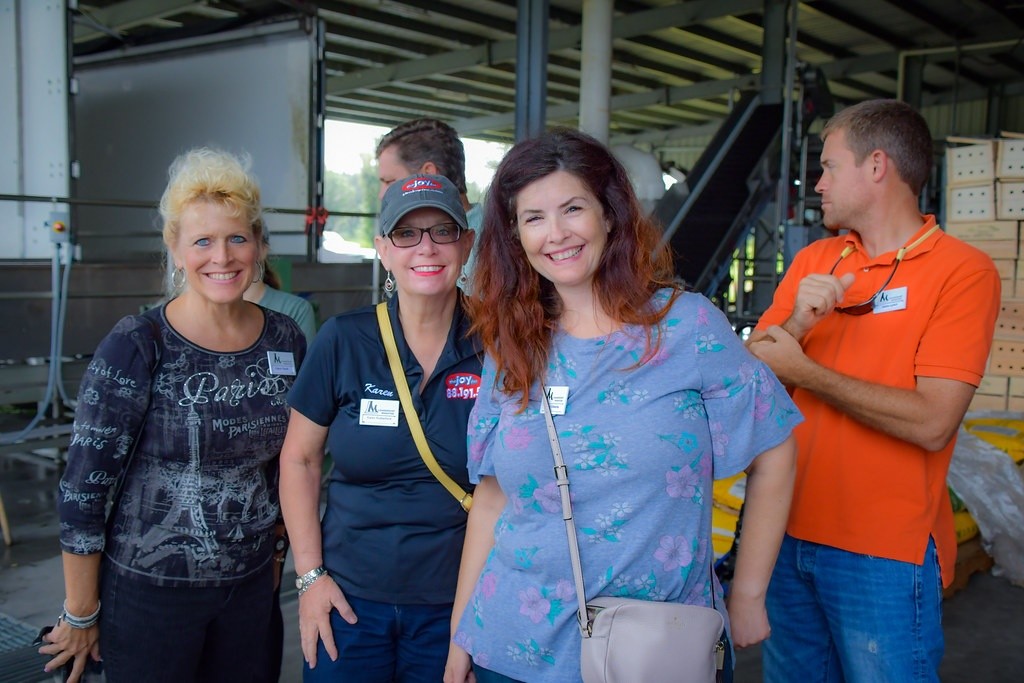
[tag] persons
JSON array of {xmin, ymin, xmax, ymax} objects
[
  {"xmin": 243, "ymin": 218, "xmax": 316, "ymax": 349},
  {"xmin": 443, "ymin": 127, "xmax": 804, "ymax": 683},
  {"xmin": 744, "ymin": 101, "xmax": 1001, "ymax": 683},
  {"xmin": 374, "ymin": 121, "xmax": 483, "ymax": 300},
  {"xmin": 278, "ymin": 170, "xmax": 486, "ymax": 683},
  {"xmin": 38, "ymin": 148, "xmax": 306, "ymax": 683}
]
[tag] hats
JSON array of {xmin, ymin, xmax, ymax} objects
[{"xmin": 378, "ymin": 174, "xmax": 468, "ymax": 241}]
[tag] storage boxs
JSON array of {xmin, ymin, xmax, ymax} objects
[{"xmin": 938, "ymin": 130, "xmax": 1024, "ymax": 419}]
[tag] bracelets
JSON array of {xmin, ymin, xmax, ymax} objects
[
  {"xmin": 297, "ymin": 571, "xmax": 327, "ymax": 596},
  {"xmin": 57, "ymin": 598, "xmax": 101, "ymax": 628}
]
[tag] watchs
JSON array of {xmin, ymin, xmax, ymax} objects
[{"xmin": 295, "ymin": 566, "xmax": 324, "ymax": 590}]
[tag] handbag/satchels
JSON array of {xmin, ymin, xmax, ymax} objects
[
  {"xmin": 33, "ymin": 615, "xmax": 103, "ymax": 683},
  {"xmin": 577, "ymin": 597, "xmax": 725, "ymax": 683}
]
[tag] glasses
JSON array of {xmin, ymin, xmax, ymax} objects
[
  {"xmin": 389, "ymin": 223, "xmax": 461, "ymax": 248},
  {"xmin": 829, "ymin": 246, "xmax": 907, "ymax": 316}
]
